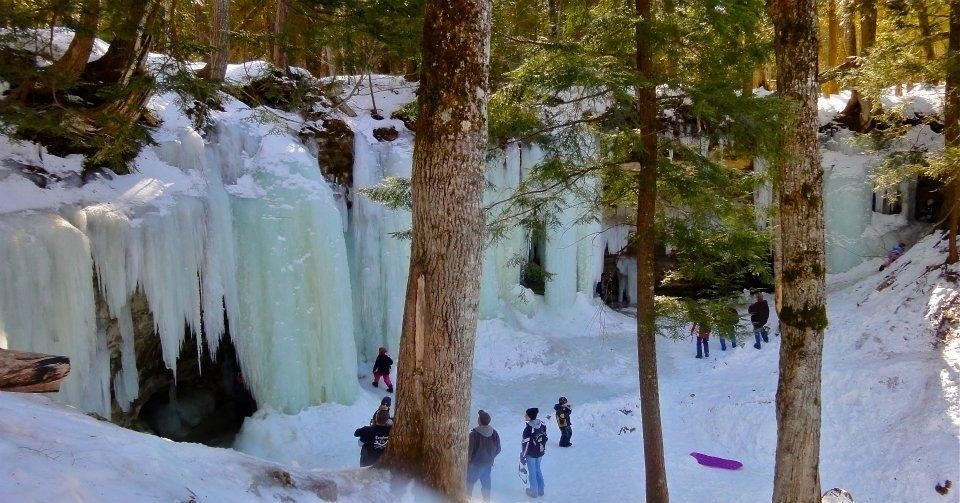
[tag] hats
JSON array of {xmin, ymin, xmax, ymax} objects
[
  {"xmin": 478, "ymin": 410, "xmax": 491, "ymax": 425},
  {"xmin": 559, "ymin": 397, "xmax": 567, "ymax": 406},
  {"xmin": 376, "ymin": 409, "xmax": 389, "ymax": 424},
  {"xmin": 380, "ymin": 397, "xmax": 392, "ymax": 404},
  {"xmin": 526, "ymin": 408, "xmax": 539, "ymax": 420}
]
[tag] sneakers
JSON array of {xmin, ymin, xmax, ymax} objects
[
  {"xmin": 387, "ymin": 387, "xmax": 393, "ymax": 392},
  {"xmin": 565, "ymin": 443, "xmax": 572, "ymax": 446},
  {"xmin": 695, "ymin": 343, "xmax": 761, "ymax": 358},
  {"xmin": 526, "ymin": 488, "xmax": 544, "ymax": 497},
  {"xmin": 372, "ymin": 381, "xmax": 378, "ymax": 387}
]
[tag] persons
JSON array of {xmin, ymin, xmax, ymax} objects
[
  {"xmin": 520, "ymin": 408, "xmax": 546, "ymax": 498},
  {"xmin": 354, "ymin": 410, "xmax": 392, "ymax": 467},
  {"xmin": 372, "ymin": 347, "xmax": 393, "ymax": 393},
  {"xmin": 748, "ymin": 292, "xmax": 769, "ymax": 349},
  {"xmin": 720, "ymin": 308, "xmax": 739, "ymax": 350},
  {"xmin": 554, "ymin": 396, "xmax": 572, "ymax": 447},
  {"xmin": 691, "ymin": 322, "xmax": 710, "ymax": 358},
  {"xmin": 879, "ymin": 243, "xmax": 905, "ymax": 271},
  {"xmin": 466, "ymin": 410, "xmax": 501, "ymax": 503},
  {"xmin": 373, "ymin": 397, "xmax": 394, "ymax": 425}
]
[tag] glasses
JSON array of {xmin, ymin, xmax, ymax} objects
[{"xmin": 381, "ymin": 403, "xmax": 390, "ymax": 407}]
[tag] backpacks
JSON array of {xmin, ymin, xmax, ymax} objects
[{"xmin": 530, "ymin": 427, "xmax": 545, "ymax": 457}]
